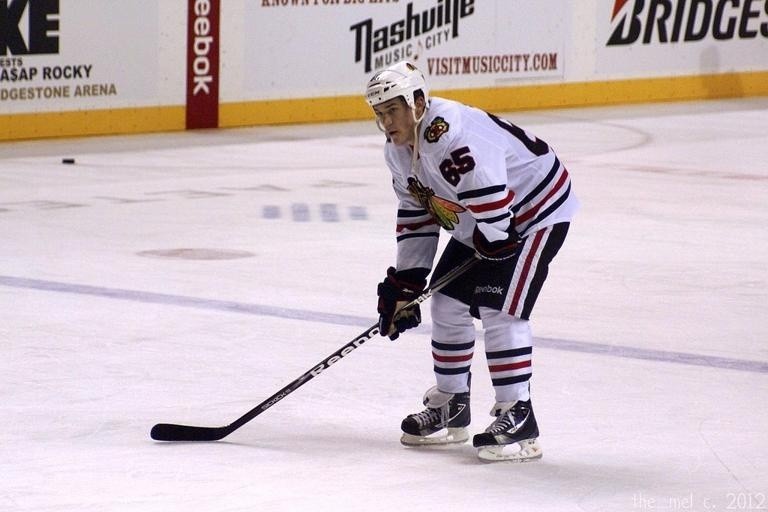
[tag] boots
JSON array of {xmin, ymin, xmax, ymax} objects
[
  {"xmin": 472, "ymin": 399, "xmax": 539, "ymax": 447},
  {"xmin": 401, "ymin": 385, "xmax": 471, "ymax": 436}
]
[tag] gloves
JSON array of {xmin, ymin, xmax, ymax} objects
[
  {"xmin": 377, "ymin": 266, "xmax": 427, "ymax": 341},
  {"xmin": 472, "ymin": 224, "xmax": 519, "ymax": 266}
]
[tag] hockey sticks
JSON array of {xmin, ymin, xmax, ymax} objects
[{"xmin": 151, "ymin": 253, "xmax": 481, "ymax": 442}]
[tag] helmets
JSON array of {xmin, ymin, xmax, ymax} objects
[{"xmin": 366, "ymin": 60, "xmax": 428, "ymax": 107}]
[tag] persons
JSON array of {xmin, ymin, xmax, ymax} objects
[{"xmin": 364, "ymin": 61, "xmax": 576, "ymax": 447}]
